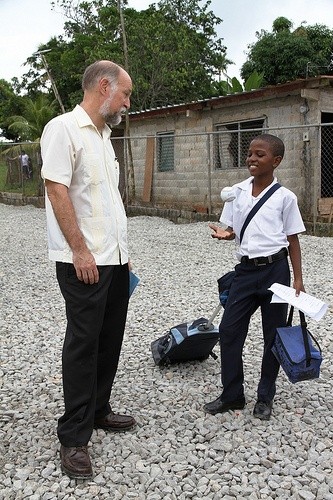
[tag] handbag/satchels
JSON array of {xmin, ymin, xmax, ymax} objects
[
  {"xmin": 217, "ymin": 271, "xmax": 236, "ymax": 310},
  {"xmin": 270, "ymin": 305, "xmax": 324, "ymax": 384}
]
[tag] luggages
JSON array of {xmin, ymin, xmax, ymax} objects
[{"xmin": 151, "ymin": 302, "xmax": 222, "ymax": 366}]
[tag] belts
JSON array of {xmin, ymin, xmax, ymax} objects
[{"xmin": 241, "ymin": 248, "xmax": 289, "ymax": 266}]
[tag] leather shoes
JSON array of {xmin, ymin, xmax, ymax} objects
[
  {"xmin": 253, "ymin": 399, "xmax": 274, "ymax": 420},
  {"xmin": 95, "ymin": 412, "xmax": 135, "ymax": 430},
  {"xmin": 203, "ymin": 396, "xmax": 246, "ymax": 414},
  {"xmin": 59, "ymin": 444, "xmax": 93, "ymax": 480}
]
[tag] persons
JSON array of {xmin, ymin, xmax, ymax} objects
[
  {"xmin": 14, "ymin": 150, "xmax": 33, "ymax": 181},
  {"xmin": 39, "ymin": 61, "xmax": 136, "ymax": 479},
  {"xmin": 203, "ymin": 134, "xmax": 306, "ymax": 421}
]
